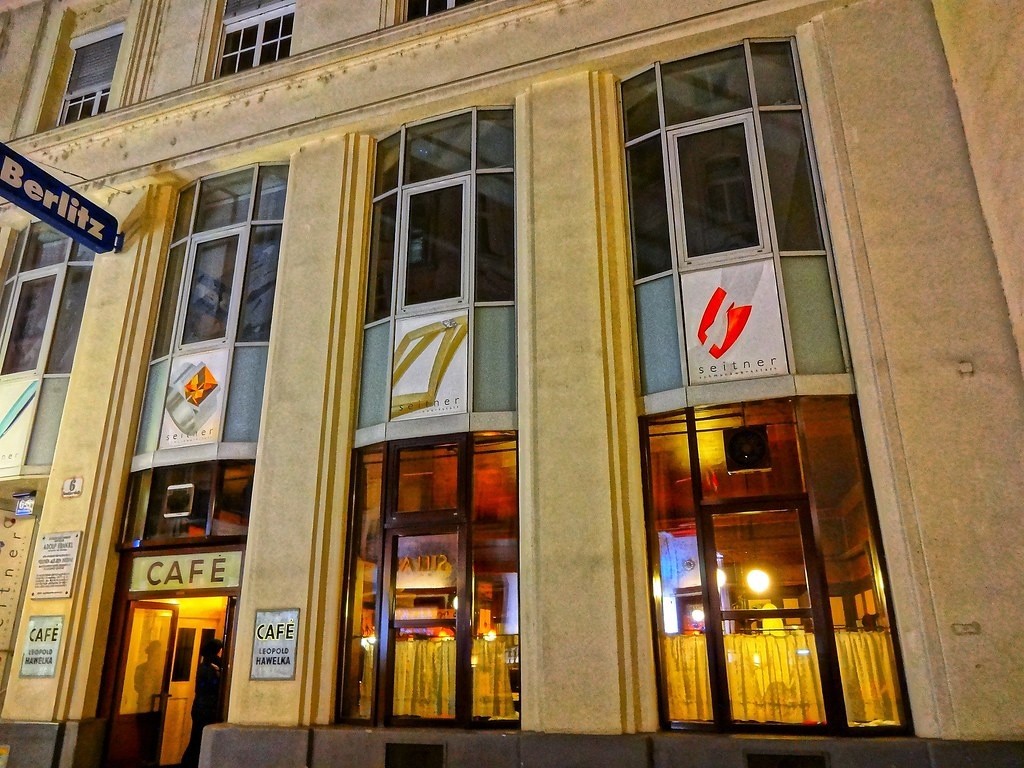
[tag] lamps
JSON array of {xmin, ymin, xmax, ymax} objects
[{"xmin": 720, "ymin": 422, "xmax": 778, "ymax": 476}]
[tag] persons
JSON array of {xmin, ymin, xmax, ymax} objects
[{"xmin": 181, "ymin": 639, "xmax": 223, "ymax": 768}]
